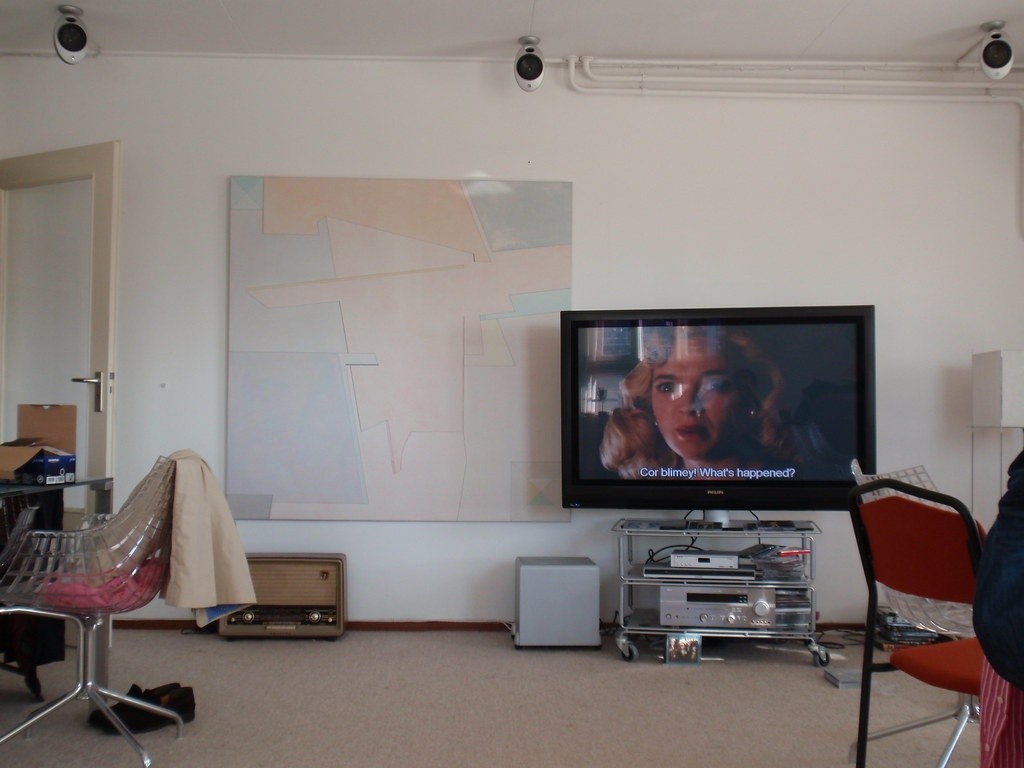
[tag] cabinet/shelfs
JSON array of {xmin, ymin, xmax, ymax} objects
[
  {"xmin": 609, "ymin": 518, "xmax": 831, "ymax": 668},
  {"xmin": 510, "ymin": 557, "xmax": 603, "ymax": 650}
]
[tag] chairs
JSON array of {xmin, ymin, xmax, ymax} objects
[
  {"xmin": 0, "ymin": 459, "xmax": 183, "ymax": 768},
  {"xmin": 851, "ymin": 458, "xmax": 984, "ymax": 768},
  {"xmin": 846, "ymin": 480, "xmax": 989, "ymax": 768}
]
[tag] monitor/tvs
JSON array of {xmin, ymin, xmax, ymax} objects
[{"xmin": 561, "ymin": 304, "xmax": 876, "ymax": 530}]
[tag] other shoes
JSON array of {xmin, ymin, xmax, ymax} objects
[{"xmin": 88, "ymin": 682, "xmax": 196, "ymax": 734}]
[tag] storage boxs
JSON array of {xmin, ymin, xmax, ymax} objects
[{"xmin": 0, "ymin": 403, "xmax": 78, "ymax": 486}]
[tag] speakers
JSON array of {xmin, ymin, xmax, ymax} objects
[{"xmin": 217, "ymin": 553, "xmax": 347, "ymax": 638}]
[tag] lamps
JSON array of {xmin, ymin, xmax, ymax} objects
[{"xmin": 972, "ymin": 348, "xmax": 1024, "ymax": 523}]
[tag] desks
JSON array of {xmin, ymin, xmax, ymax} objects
[{"xmin": 0, "ymin": 478, "xmax": 114, "ymax": 722}]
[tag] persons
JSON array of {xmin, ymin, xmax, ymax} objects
[
  {"xmin": 598, "ymin": 326, "xmax": 798, "ymax": 471},
  {"xmin": 971, "ymin": 449, "xmax": 1024, "ymax": 768}
]
